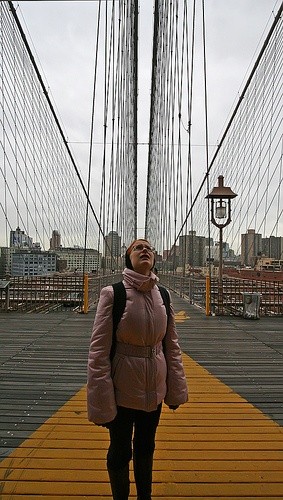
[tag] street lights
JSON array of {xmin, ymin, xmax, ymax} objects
[{"xmin": 206, "ymin": 176, "xmax": 236, "ymax": 304}]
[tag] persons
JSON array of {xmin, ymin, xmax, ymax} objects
[{"xmin": 84, "ymin": 237, "xmax": 192, "ymax": 500}]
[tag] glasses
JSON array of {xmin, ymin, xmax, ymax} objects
[{"xmin": 128, "ymin": 244, "xmax": 155, "ymax": 256}]
